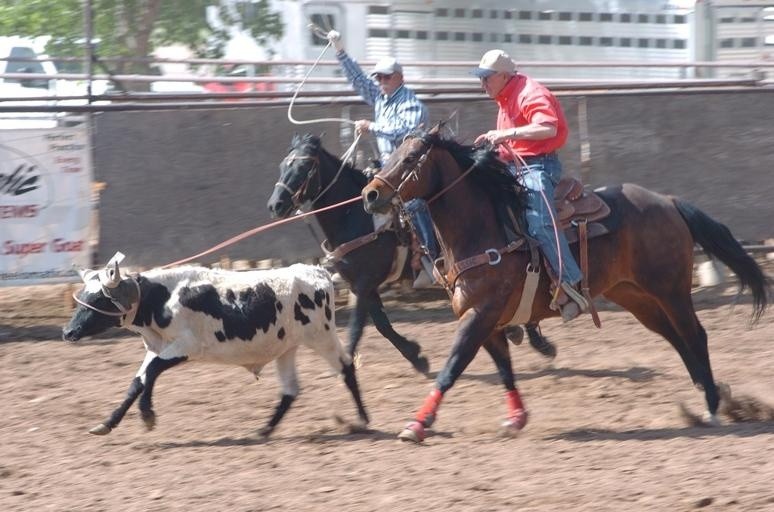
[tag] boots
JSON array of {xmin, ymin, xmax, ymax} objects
[
  {"xmin": 561, "ymin": 302, "xmax": 578, "ymax": 323},
  {"xmin": 412, "ymin": 270, "xmax": 432, "ymax": 288}
]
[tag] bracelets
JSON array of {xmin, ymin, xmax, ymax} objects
[{"xmin": 511, "ymin": 127, "xmax": 516, "ymax": 137}]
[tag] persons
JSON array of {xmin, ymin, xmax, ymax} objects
[
  {"xmin": 326, "ymin": 30, "xmax": 444, "ymax": 290},
  {"xmin": 474, "ymin": 49, "xmax": 583, "ymax": 323}
]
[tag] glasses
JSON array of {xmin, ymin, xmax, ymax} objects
[{"xmin": 375, "ymin": 76, "xmax": 391, "ymax": 81}]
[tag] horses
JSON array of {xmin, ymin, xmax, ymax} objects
[
  {"xmin": 266, "ymin": 128, "xmax": 559, "ymax": 375},
  {"xmin": 360, "ymin": 117, "xmax": 774, "ymax": 445}
]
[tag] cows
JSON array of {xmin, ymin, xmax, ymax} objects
[{"xmin": 61, "ymin": 256, "xmax": 371, "ymax": 441}]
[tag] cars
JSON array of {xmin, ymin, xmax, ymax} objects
[{"xmin": 0, "ymin": 35, "xmax": 280, "ymax": 127}]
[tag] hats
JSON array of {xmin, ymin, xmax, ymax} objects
[
  {"xmin": 469, "ymin": 49, "xmax": 514, "ymax": 77},
  {"xmin": 371, "ymin": 57, "xmax": 402, "ymax": 77}
]
